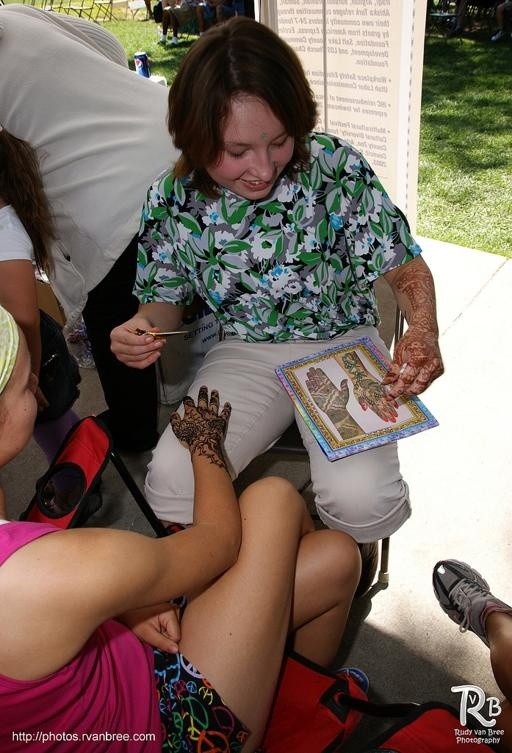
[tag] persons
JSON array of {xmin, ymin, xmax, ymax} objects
[
  {"xmin": 1, "ymin": 3, "xmax": 184, "ymax": 452},
  {"xmin": 0, "ymin": 305, "xmax": 370, "ymax": 752},
  {"xmin": 334, "ymin": 351, "xmax": 399, "ymax": 422},
  {"xmin": 0, "ymin": 128, "xmax": 104, "ymax": 527},
  {"xmin": 432, "ymin": 560, "xmax": 511, "ymax": 753},
  {"xmin": 110, "ymin": 16, "xmax": 444, "ymax": 597},
  {"xmin": 305, "ymin": 367, "xmax": 365, "ymax": 440}
]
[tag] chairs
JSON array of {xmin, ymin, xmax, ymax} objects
[{"xmin": 18, "ymin": 413, "xmax": 506, "ymax": 753}]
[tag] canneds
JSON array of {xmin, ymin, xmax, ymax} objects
[{"xmin": 133, "ymin": 51, "xmax": 150, "ymax": 78}]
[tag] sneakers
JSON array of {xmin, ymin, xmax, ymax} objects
[
  {"xmin": 161, "ymin": 369, "xmax": 195, "ymax": 405},
  {"xmin": 432, "ymin": 559, "xmax": 512, "ymax": 648}
]
